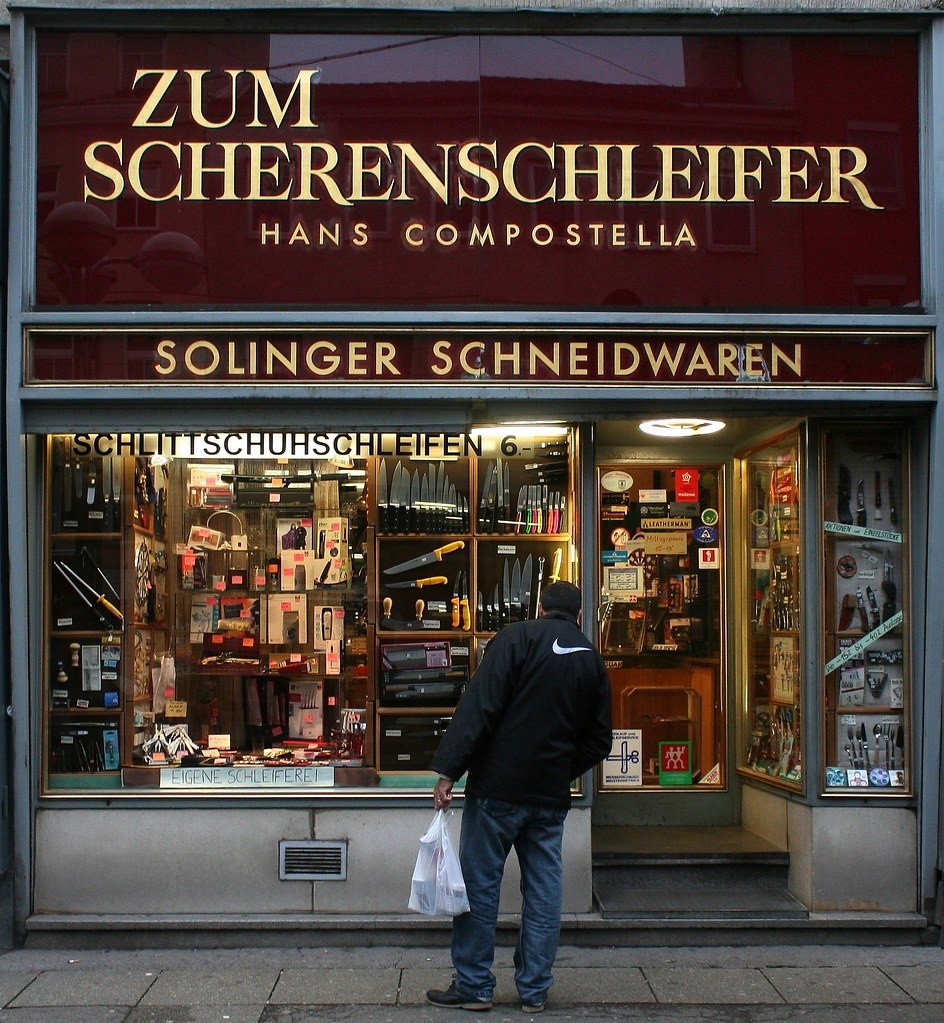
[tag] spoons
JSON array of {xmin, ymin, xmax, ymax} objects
[
  {"xmin": 855, "ymin": 729, "xmax": 865, "ymax": 770},
  {"xmin": 847, "ymin": 723, "xmax": 859, "ymax": 770},
  {"xmin": 844, "ymin": 742, "xmax": 853, "ymax": 767},
  {"xmin": 872, "ymin": 723, "xmax": 883, "ymax": 769}
]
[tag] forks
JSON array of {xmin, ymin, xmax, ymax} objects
[
  {"xmin": 889, "ymin": 728, "xmax": 897, "ymax": 770},
  {"xmin": 882, "ymin": 724, "xmax": 892, "ymax": 770}
]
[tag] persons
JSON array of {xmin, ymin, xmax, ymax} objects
[{"xmin": 427, "ymin": 581, "xmax": 612, "ymax": 1013}]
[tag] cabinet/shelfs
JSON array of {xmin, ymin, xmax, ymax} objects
[{"xmin": 50, "ymin": 432, "xmax": 906, "ymax": 792}]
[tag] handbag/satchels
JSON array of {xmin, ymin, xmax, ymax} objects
[{"xmin": 407, "ymin": 808, "xmax": 471, "ymax": 918}]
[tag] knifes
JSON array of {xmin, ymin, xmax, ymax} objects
[
  {"xmin": 378, "ymin": 438, "xmax": 569, "ymax": 736},
  {"xmin": 836, "ymin": 459, "xmax": 906, "ymax": 709},
  {"xmin": 860, "ymin": 721, "xmax": 872, "ymax": 771}
]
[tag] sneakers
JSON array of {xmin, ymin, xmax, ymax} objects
[
  {"xmin": 425, "ymin": 981, "xmax": 493, "ymax": 1010},
  {"xmin": 521, "ymin": 992, "xmax": 549, "ymax": 1012}
]
